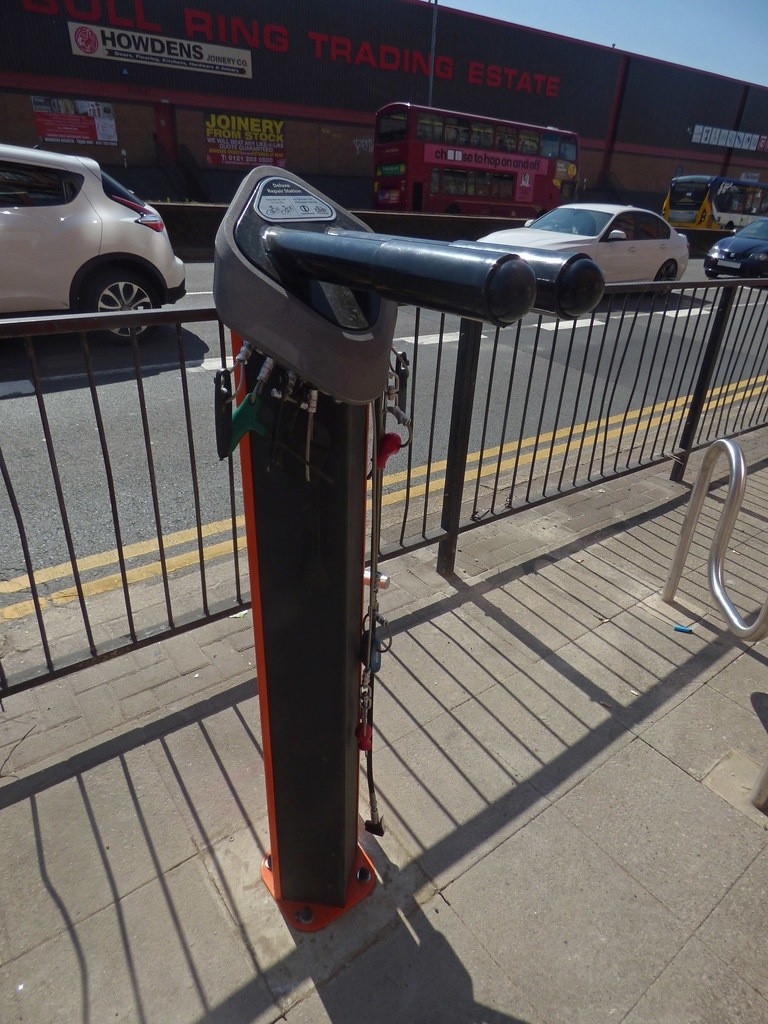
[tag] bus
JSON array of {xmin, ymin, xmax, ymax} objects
[
  {"xmin": 661, "ymin": 175, "xmax": 768, "ymax": 232},
  {"xmin": 371, "ymin": 102, "xmax": 587, "ymax": 218}
]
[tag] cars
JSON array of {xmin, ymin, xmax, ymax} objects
[
  {"xmin": 0, "ymin": 144, "xmax": 186, "ymax": 346},
  {"xmin": 476, "ymin": 203, "xmax": 690, "ymax": 298},
  {"xmin": 703, "ymin": 218, "xmax": 768, "ymax": 289}
]
